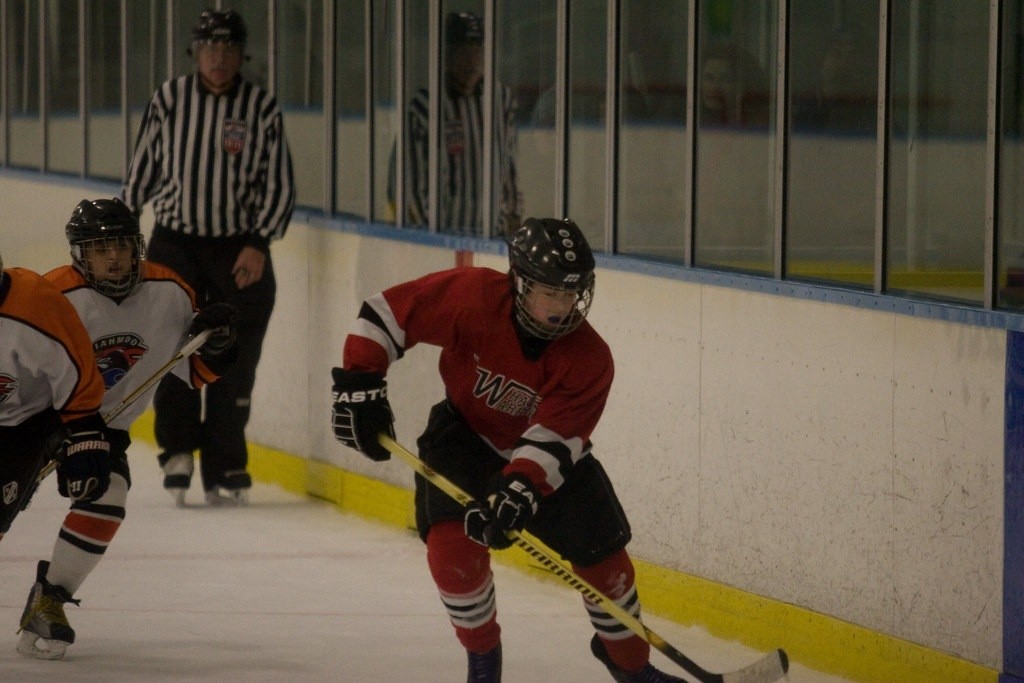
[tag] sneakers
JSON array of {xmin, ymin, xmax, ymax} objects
[
  {"xmin": 163, "ymin": 448, "xmax": 194, "ymax": 506},
  {"xmin": 204, "ymin": 469, "xmax": 252, "ymax": 508},
  {"xmin": 591, "ymin": 631, "xmax": 688, "ymax": 683},
  {"xmin": 15, "ymin": 561, "xmax": 82, "ymax": 661},
  {"xmin": 467, "ymin": 640, "xmax": 502, "ymax": 683}
]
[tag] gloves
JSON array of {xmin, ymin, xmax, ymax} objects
[
  {"xmin": 60, "ymin": 410, "xmax": 111, "ymax": 502},
  {"xmin": 190, "ymin": 306, "xmax": 237, "ymax": 357},
  {"xmin": 464, "ymin": 474, "xmax": 544, "ymax": 549},
  {"xmin": 331, "ymin": 367, "xmax": 396, "ymax": 460}
]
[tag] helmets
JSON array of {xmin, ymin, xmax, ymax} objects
[
  {"xmin": 445, "ymin": 10, "xmax": 485, "ymax": 43},
  {"xmin": 509, "ymin": 217, "xmax": 596, "ymax": 341},
  {"xmin": 65, "ymin": 197, "xmax": 147, "ymax": 297},
  {"xmin": 192, "ymin": 9, "xmax": 246, "ymax": 46}
]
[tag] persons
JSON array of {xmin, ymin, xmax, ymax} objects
[
  {"xmin": 388, "ymin": 13, "xmax": 524, "ymax": 236},
  {"xmin": 0, "ymin": 255, "xmax": 112, "ymax": 542},
  {"xmin": 330, "ymin": 217, "xmax": 688, "ymax": 683},
  {"xmin": 584, "ymin": 33, "xmax": 896, "ymax": 132},
  {"xmin": 119, "ymin": 8, "xmax": 297, "ymax": 507},
  {"xmin": 16, "ymin": 197, "xmax": 240, "ymax": 661}
]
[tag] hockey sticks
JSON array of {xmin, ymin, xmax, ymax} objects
[
  {"xmin": 22, "ymin": 324, "xmax": 218, "ymax": 510},
  {"xmin": 376, "ymin": 431, "xmax": 790, "ymax": 683}
]
